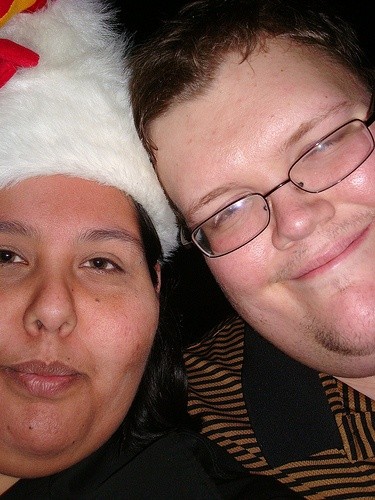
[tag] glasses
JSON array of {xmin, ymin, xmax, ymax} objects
[{"xmin": 180, "ymin": 109, "xmax": 375, "ymax": 258}]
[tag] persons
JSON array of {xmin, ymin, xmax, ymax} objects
[
  {"xmin": 0, "ymin": 0, "xmax": 305, "ymax": 500},
  {"xmin": 130, "ymin": 0, "xmax": 375, "ymax": 500}
]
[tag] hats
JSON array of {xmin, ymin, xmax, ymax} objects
[{"xmin": 0, "ymin": 0, "xmax": 180, "ymax": 261}]
